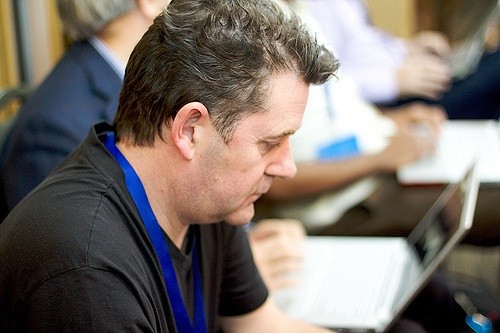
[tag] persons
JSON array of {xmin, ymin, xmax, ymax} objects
[
  {"xmin": 0, "ymin": 0, "xmax": 308, "ymax": 287},
  {"xmin": 0, "ymin": 0, "xmax": 343, "ymax": 333},
  {"xmin": 252, "ymin": 77, "xmax": 500, "ymax": 333},
  {"xmin": 284, "ymin": 0, "xmax": 500, "ymax": 119}
]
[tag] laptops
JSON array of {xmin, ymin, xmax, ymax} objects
[
  {"xmin": 266, "ymin": 152, "xmax": 481, "ymax": 333},
  {"xmin": 397, "ymin": 119, "xmax": 500, "ymax": 187}
]
